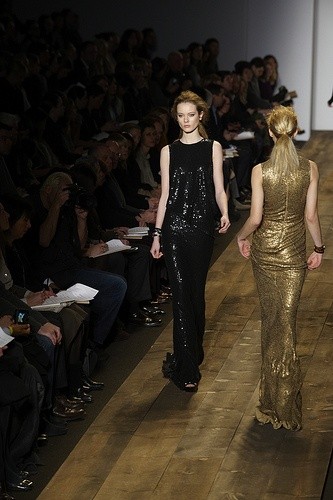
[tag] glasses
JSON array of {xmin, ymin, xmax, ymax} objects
[{"xmin": 113, "ymin": 153, "xmax": 121, "ymax": 157}]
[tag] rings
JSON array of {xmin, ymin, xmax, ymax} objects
[{"xmin": 64, "ymin": 191, "xmax": 67, "ymax": 194}]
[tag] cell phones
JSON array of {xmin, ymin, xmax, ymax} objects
[{"xmin": 13, "ymin": 309, "xmax": 29, "ymax": 325}]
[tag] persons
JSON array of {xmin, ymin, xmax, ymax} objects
[
  {"xmin": 0, "ymin": 7, "xmax": 305, "ymax": 500},
  {"xmin": 237, "ymin": 109, "xmax": 325, "ymax": 429},
  {"xmin": 328, "ymin": 96, "xmax": 333, "ymax": 105}
]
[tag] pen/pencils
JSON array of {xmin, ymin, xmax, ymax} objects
[
  {"xmin": 98, "ymin": 235, "xmax": 101, "ymax": 244},
  {"xmin": 135, "ymin": 211, "xmax": 142, "ymax": 218},
  {"xmin": 41, "ymin": 287, "xmax": 47, "ymax": 293}
]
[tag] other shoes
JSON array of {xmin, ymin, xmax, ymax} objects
[
  {"xmin": 233, "ymin": 186, "xmax": 251, "ymax": 210},
  {"xmin": 18, "ymin": 420, "xmax": 68, "ymax": 486},
  {"xmin": 185, "ymin": 382, "xmax": 198, "ymax": 393}
]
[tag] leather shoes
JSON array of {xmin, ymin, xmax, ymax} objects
[
  {"xmin": 4, "ymin": 478, "xmax": 32, "ymax": 492},
  {"xmin": 0, "ymin": 492, "xmax": 16, "ymax": 500},
  {"xmin": 122, "ymin": 307, "xmax": 165, "ymax": 327},
  {"xmin": 150, "ymin": 285, "xmax": 177, "ymax": 307},
  {"xmin": 51, "ymin": 404, "xmax": 86, "ymax": 422},
  {"xmin": 56, "ymin": 395, "xmax": 86, "ymax": 409},
  {"xmin": 80, "ymin": 377, "xmax": 105, "ymax": 390},
  {"xmin": 71, "ymin": 386, "xmax": 95, "ymax": 402}
]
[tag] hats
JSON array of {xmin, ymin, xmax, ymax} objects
[{"xmin": 250, "ymin": 57, "xmax": 264, "ymax": 66}]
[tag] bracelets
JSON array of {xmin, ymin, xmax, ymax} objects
[
  {"xmin": 7, "ymin": 326, "xmax": 13, "ymax": 336},
  {"xmin": 151, "ymin": 228, "xmax": 163, "ymax": 237},
  {"xmin": 314, "ymin": 245, "xmax": 325, "ymax": 253}
]
